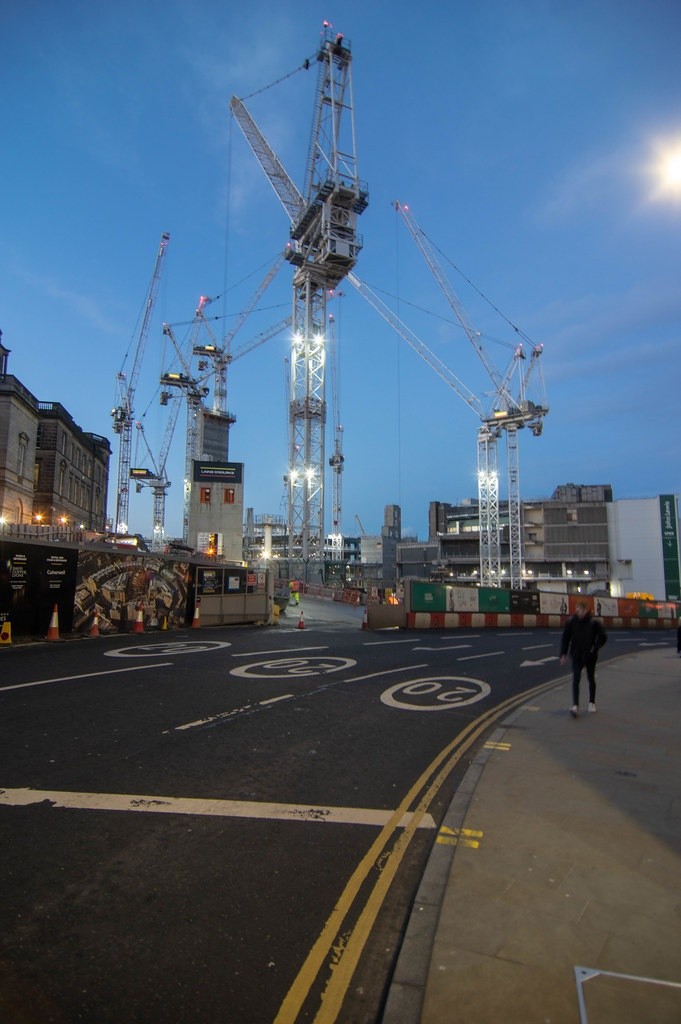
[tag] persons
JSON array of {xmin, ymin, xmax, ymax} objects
[
  {"xmin": 559, "ymin": 600, "xmax": 607, "ymax": 719},
  {"xmin": 288, "ymin": 577, "xmax": 300, "ymax": 606},
  {"xmin": 676, "ymin": 616, "xmax": 681, "ymax": 656}
]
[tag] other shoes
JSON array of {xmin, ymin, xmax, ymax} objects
[
  {"xmin": 587, "ymin": 703, "xmax": 597, "ymax": 713},
  {"xmin": 569, "ymin": 704, "xmax": 579, "ymax": 718}
]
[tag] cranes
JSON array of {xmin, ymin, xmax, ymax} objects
[
  {"xmin": 343, "ymin": 196, "xmax": 552, "ymax": 590},
  {"xmin": 227, "ymin": 16, "xmax": 374, "ymax": 587},
  {"xmin": 113, "ymin": 230, "xmax": 340, "ymax": 555}
]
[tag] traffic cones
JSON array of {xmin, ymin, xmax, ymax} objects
[
  {"xmin": 189, "ymin": 602, "xmax": 203, "ymax": 630},
  {"xmin": 293, "ymin": 610, "xmax": 308, "ymax": 629},
  {"xmin": 37, "ymin": 603, "xmax": 66, "ymax": 643},
  {"xmin": 359, "ymin": 608, "xmax": 370, "ymax": 632},
  {"xmin": 0, "ymin": 621, "xmax": 13, "ymax": 647},
  {"xmin": 161, "ymin": 616, "xmax": 168, "ymax": 631},
  {"xmin": 129, "ymin": 603, "xmax": 146, "ymax": 635},
  {"xmin": 83, "ymin": 610, "xmax": 103, "ymax": 638}
]
